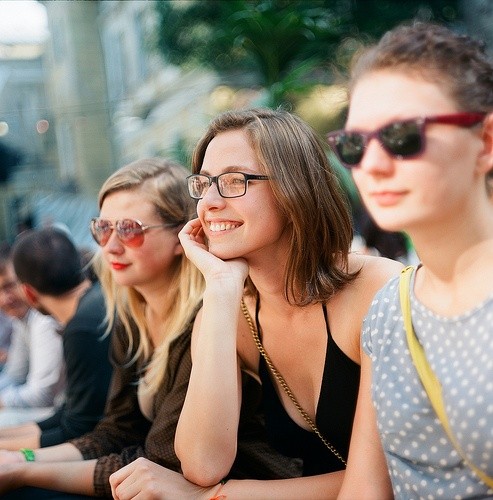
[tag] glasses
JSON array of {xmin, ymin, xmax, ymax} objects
[
  {"xmin": 325, "ymin": 113, "xmax": 487, "ymax": 168},
  {"xmin": 89, "ymin": 217, "xmax": 182, "ymax": 248},
  {"xmin": 186, "ymin": 171, "xmax": 279, "ymax": 200}
]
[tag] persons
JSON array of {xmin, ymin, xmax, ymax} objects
[
  {"xmin": 335, "ymin": 18, "xmax": 492, "ymax": 500},
  {"xmin": 0, "ymin": 220, "xmax": 120, "ymax": 450},
  {"xmin": 109, "ymin": 108, "xmax": 407, "ymax": 500},
  {"xmin": 0, "ymin": 157, "xmax": 205, "ymax": 500}
]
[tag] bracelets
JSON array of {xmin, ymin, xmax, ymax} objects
[
  {"xmin": 209, "ymin": 476, "xmax": 232, "ymax": 500},
  {"xmin": 19, "ymin": 447, "xmax": 36, "ymax": 464}
]
